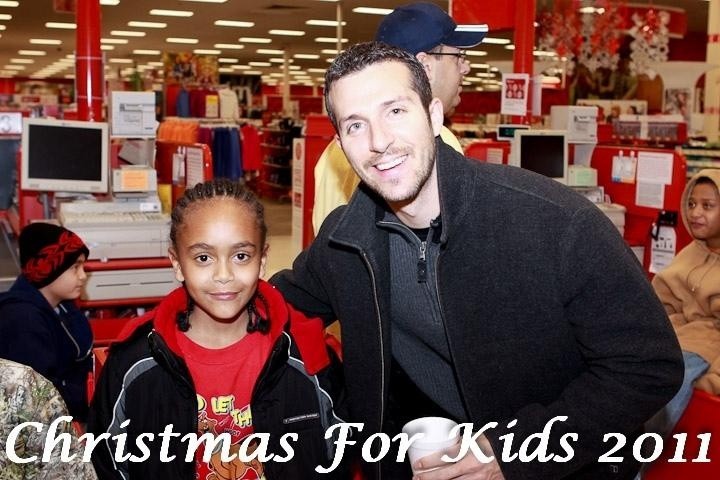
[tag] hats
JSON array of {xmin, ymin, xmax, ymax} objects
[
  {"xmin": 376, "ymin": 2, "xmax": 489, "ymax": 57},
  {"xmin": 18, "ymin": 222, "xmax": 89, "ymax": 291}
]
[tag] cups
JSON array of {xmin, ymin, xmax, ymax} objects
[{"xmin": 403, "ymin": 415, "xmax": 460, "ymax": 479}]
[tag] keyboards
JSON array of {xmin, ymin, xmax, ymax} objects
[{"xmin": 59, "ymin": 211, "xmax": 167, "ymax": 228}]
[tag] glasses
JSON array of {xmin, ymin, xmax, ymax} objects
[{"xmin": 425, "ymin": 51, "xmax": 467, "ymax": 64}]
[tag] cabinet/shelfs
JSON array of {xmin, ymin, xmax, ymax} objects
[
  {"xmin": 73, "ymin": 255, "xmax": 178, "ymax": 351},
  {"xmin": 258, "ymin": 124, "xmax": 294, "ymax": 191}
]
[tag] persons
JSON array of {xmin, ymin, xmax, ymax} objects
[
  {"xmin": 87, "ymin": 181, "xmax": 357, "ymax": 480},
  {"xmin": 267, "ymin": 42, "xmax": 685, "ymax": 480},
  {"xmin": 0, "ymin": 223, "xmax": 93, "ymax": 425},
  {"xmin": 634, "ymin": 169, "xmax": 720, "ymax": 480},
  {"xmin": 312, "ymin": 1, "xmax": 487, "ymax": 237}
]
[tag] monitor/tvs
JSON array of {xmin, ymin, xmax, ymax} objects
[
  {"xmin": 514, "ymin": 129, "xmax": 569, "ymax": 186},
  {"xmin": 21, "ymin": 117, "xmax": 109, "ymax": 193}
]
